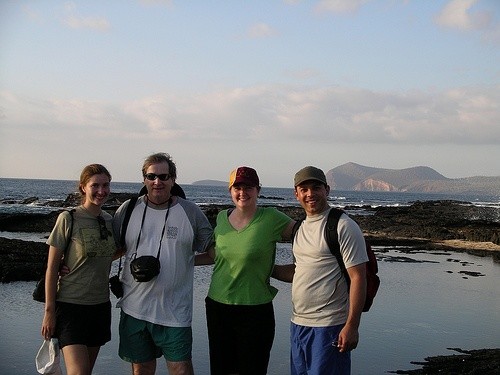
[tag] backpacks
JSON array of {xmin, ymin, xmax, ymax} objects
[{"xmin": 290, "ymin": 208, "xmax": 380, "ymax": 311}]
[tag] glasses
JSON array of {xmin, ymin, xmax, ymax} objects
[{"xmin": 144, "ymin": 173, "xmax": 173, "ymax": 181}]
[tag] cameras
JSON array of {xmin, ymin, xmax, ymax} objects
[{"xmin": 130, "ymin": 256, "xmax": 160, "ymax": 282}]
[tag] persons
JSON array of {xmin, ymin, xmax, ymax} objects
[
  {"xmin": 41, "ymin": 164, "xmax": 118, "ymax": 375},
  {"xmin": 195, "ymin": 166, "xmax": 296, "ymax": 375},
  {"xmin": 273, "ymin": 166, "xmax": 369, "ymax": 375},
  {"xmin": 59, "ymin": 153, "xmax": 214, "ymax": 375}
]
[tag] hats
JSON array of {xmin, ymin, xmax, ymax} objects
[
  {"xmin": 228, "ymin": 167, "xmax": 259, "ymax": 188},
  {"xmin": 294, "ymin": 166, "xmax": 327, "ymax": 186},
  {"xmin": 35, "ymin": 338, "xmax": 62, "ymax": 375}
]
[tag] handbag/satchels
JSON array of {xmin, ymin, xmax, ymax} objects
[{"xmin": 32, "ymin": 278, "xmax": 45, "ymax": 303}]
